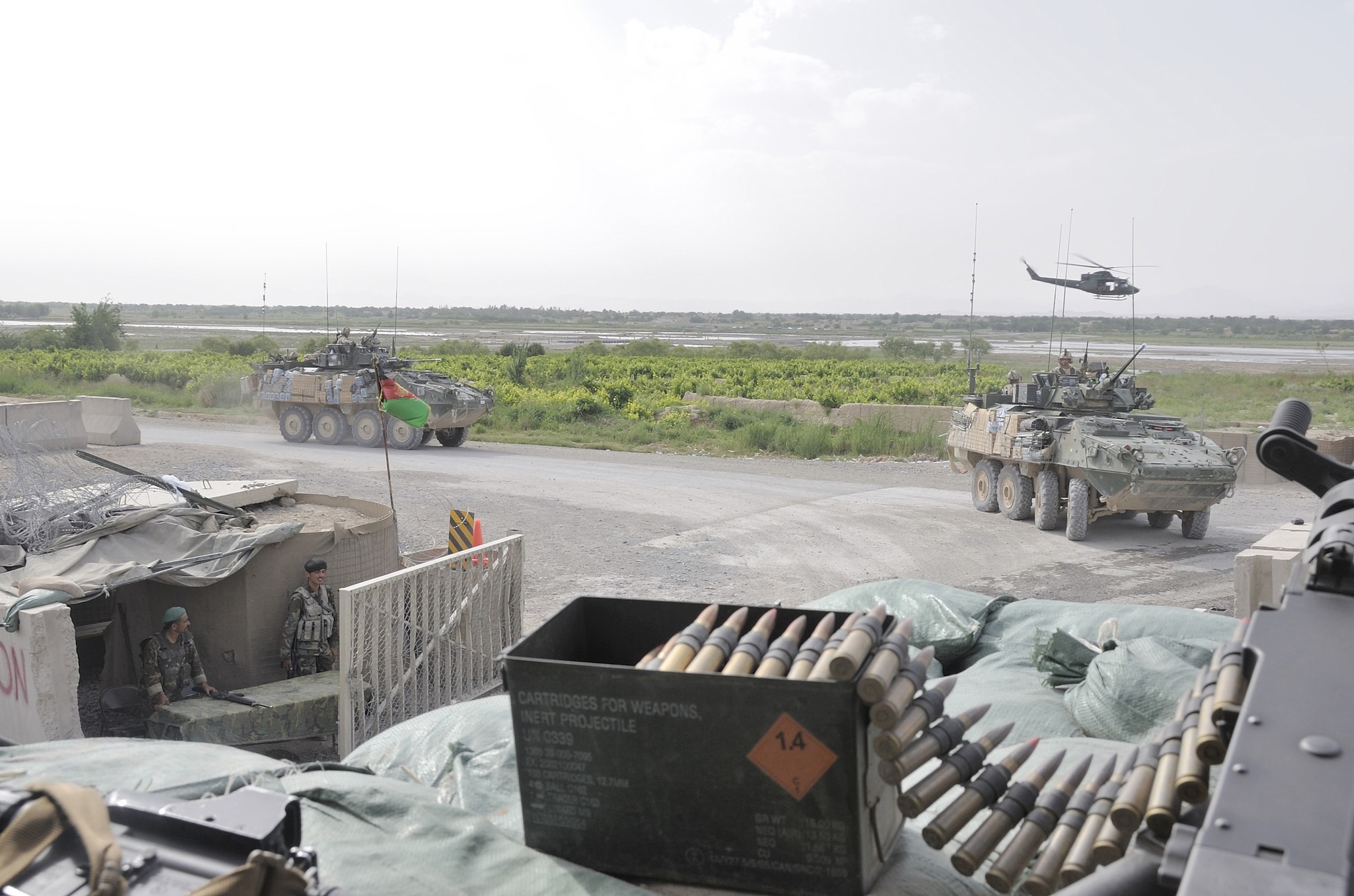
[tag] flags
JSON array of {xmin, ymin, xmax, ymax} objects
[{"xmin": 374, "ymin": 357, "xmax": 431, "ymax": 428}]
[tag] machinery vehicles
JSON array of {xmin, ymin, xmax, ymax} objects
[
  {"xmin": 943, "ymin": 342, "xmax": 1247, "ymax": 543},
  {"xmin": 241, "ymin": 322, "xmax": 496, "ymax": 451}
]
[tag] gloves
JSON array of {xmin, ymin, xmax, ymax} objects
[{"xmin": 352, "ymin": 341, "xmax": 356, "ymax": 347}]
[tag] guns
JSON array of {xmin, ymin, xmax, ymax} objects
[
  {"xmin": 191, "ymin": 685, "xmax": 274, "ymax": 710},
  {"xmin": 281, "ymin": 635, "xmax": 299, "ymax": 679}
]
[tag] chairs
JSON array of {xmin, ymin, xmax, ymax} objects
[{"xmin": 100, "ymin": 684, "xmax": 155, "ymax": 738}]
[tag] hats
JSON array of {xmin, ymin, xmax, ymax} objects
[
  {"xmin": 163, "ymin": 606, "xmax": 186, "ymax": 623},
  {"xmin": 305, "ymin": 559, "xmax": 327, "ymax": 572}
]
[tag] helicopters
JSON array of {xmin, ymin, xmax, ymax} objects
[{"xmin": 1019, "ymin": 251, "xmax": 1161, "ymax": 302}]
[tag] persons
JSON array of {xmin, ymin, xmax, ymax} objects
[
  {"xmin": 1000, "ymin": 369, "xmax": 1024, "ymax": 394},
  {"xmin": 1049, "ymin": 349, "xmax": 1081, "ymax": 374},
  {"xmin": 283, "ymin": 327, "xmax": 370, "ymax": 361},
  {"xmin": 279, "ymin": 558, "xmax": 339, "ymax": 677},
  {"xmin": 137, "ymin": 606, "xmax": 218, "ymax": 739}
]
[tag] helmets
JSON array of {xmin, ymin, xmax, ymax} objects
[
  {"xmin": 1008, "ymin": 370, "xmax": 1018, "ymax": 380},
  {"xmin": 304, "ymin": 354, "xmax": 312, "ymax": 359},
  {"xmin": 284, "ymin": 355, "xmax": 292, "ymax": 360},
  {"xmin": 1060, "ymin": 351, "xmax": 1072, "ymax": 360},
  {"xmin": 341, "ymin": 327, "xmax": 350, "ymax": 333},
  {"xmin": 290, "ymin": 351, "xmax": 299, "ymax": 358}
]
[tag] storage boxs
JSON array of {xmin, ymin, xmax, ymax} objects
[{"xmin": 496, "ymin": 597, "xmax": 909, "ymax": 896}]
